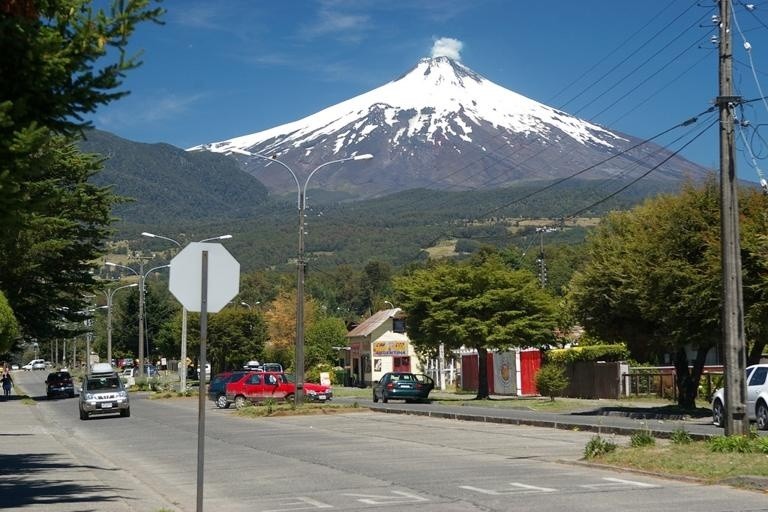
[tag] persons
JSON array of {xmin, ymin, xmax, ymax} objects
[
  {"xmin": 6, "ymin": 373, "xmax": 15, "ymax": 400},
  {"xmin": 0, "ymin": 374, "xmax": 7, "ymax": 395}
]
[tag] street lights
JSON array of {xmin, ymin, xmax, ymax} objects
[
  {"xmin": 105, "ymin": 262, "xmax": 170, "ymax": 377},
  {"xmin": 141, "ymin": 232, "xmax": 232, "ymax": 392},
  {"xmin": 78, "ymin": 305, "xmax": 108, "ymax": 368},
  {"xmin": 231, "ymin": 149, "xmax": 373, "ymax": 405},
  {"xmin": 98, "ymin": 284, "xmax": 138, "ymax": 366}
]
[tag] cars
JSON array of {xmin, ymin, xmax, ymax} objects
[
  {"xmin": 208, "ymin": 360, "xmax": 332, "ymax": 409},
  {"xmin": 22, "ymin": 359, "xmax": 52, "ymax": 371},
  {"xmin": 112, "ymin": 358, "xmax": 159, "ymax": 375},
  {"xmin": 713, "ymin": 364, "xmax": 768, "ymax": 429},
  {"xmin": 45, "ymin": 372, "xmax": 74, "ymax": 398},
  {"xmin": 373, "ymin": 372, "xmax": 434, "ymax": 403},
  {"xmin": 77, "ymin": 372, "xmax": 130, "ymax": 419}
]
